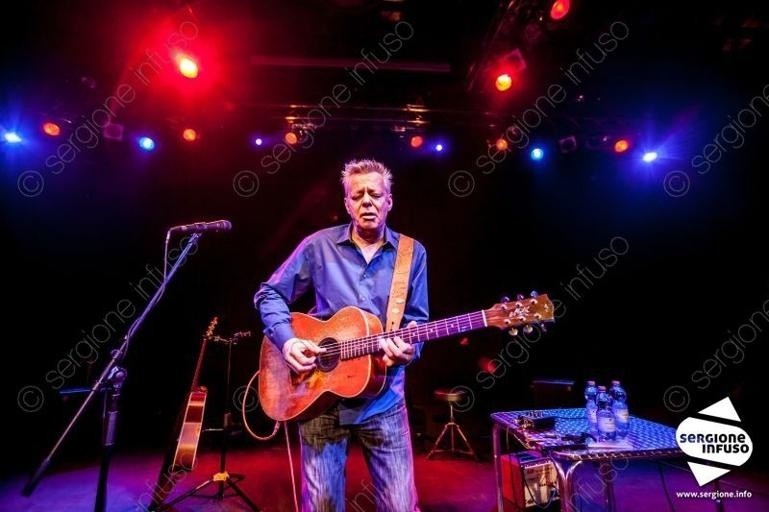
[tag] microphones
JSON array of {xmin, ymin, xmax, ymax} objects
[{"xmin": 173, "ymin": 219, "xmax": 233, "ymax": 235}]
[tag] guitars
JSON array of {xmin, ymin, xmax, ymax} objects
[
  {"xmin": 173, "ymin": 317, "xmax": 217, "ymax": 472},
  {"xmin": 259, "ymin": 291, "xmax": 555, "ymax": 424}
]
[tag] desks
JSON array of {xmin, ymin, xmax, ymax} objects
[{"xmin": 490, "ymin": 407, "xmax": 724, "ymax": 511}]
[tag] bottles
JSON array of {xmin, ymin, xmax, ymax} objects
[
  {"xmin": 584, "ymin": 380, "xmax": 598, "ymax": 424},
  {"xmin": 610, "ymin": 380, "xmax": 631, "ymax": 436},
  {"xmin": 594, "ymin": 385, "xmax": 615, "ymax": 438}
]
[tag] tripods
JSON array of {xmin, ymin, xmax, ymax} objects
[{"xmin": 157, "ymin": 330, "xmax": 258, "ymax": 512}]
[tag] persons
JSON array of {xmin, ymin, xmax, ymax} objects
[{"xmin": 254, "ymin": 159, "xmax": 428, "ymax": 512}]
